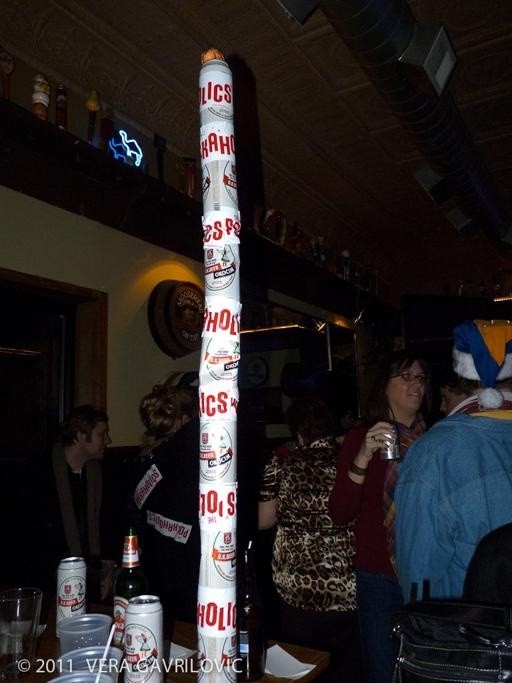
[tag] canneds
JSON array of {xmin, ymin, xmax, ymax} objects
[
  {"xmin": 55, "ymin": 557, "xmax": 89, "ymax": 638},
  {"xmin": 192, "ymin": 59, "xmax": 243, "ymax": 683},
  {"xmin": 380, "ymin": 430, "xmax": 401, "ymax": 459},
  {"xmin": 123, "ymin": 594, "xmax": 168, "ymax": 683}
]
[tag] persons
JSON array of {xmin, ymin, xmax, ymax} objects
[
  {"xmin": 1, "ymin": 345, "xmax": 508, "ymax": 679},
  {"xmin": 391, "ymin": 315, "xmax": 509, "ymax": 598}
]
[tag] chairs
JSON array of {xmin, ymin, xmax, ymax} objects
[{"xmin": 237, "ymin": 527, "xmax": 278, "ymax": 634}]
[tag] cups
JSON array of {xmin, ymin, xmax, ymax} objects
[
  {"xmin": 48, "ymin": 612, "xmax": 123, "ymax": 683},
  {"xmin": 0, "ymin": 586, "xmax": 43, "ymax": 683}
]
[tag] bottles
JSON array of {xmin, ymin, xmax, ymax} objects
[
  {"xmin": 113, "ymin": 524, "xmax": 146, "ymax": 647},
  {"xmin": 288, "ymin": 221, "xmax": 327, "ymax": 269},
  {"xmin": 235, "ymin": 571, "xmax": 266, "ymax": 682}
]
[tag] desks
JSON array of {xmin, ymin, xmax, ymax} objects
[{"xmin": 1, "ymin": 593, "xmax": 338, "ymax": 682}]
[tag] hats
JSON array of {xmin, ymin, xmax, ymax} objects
[{"xmin": 452, "ymin": 320, "xmax": 512, "ymax": 409}]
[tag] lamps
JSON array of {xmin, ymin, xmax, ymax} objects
[{"xmin": 311, "ymin": 317, "xmax": 327, "ymax": 333}]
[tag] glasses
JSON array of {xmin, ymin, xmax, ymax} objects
[{"xmin": 389, "ymin": 374, "xmax": 425, "ymax": 383}]
